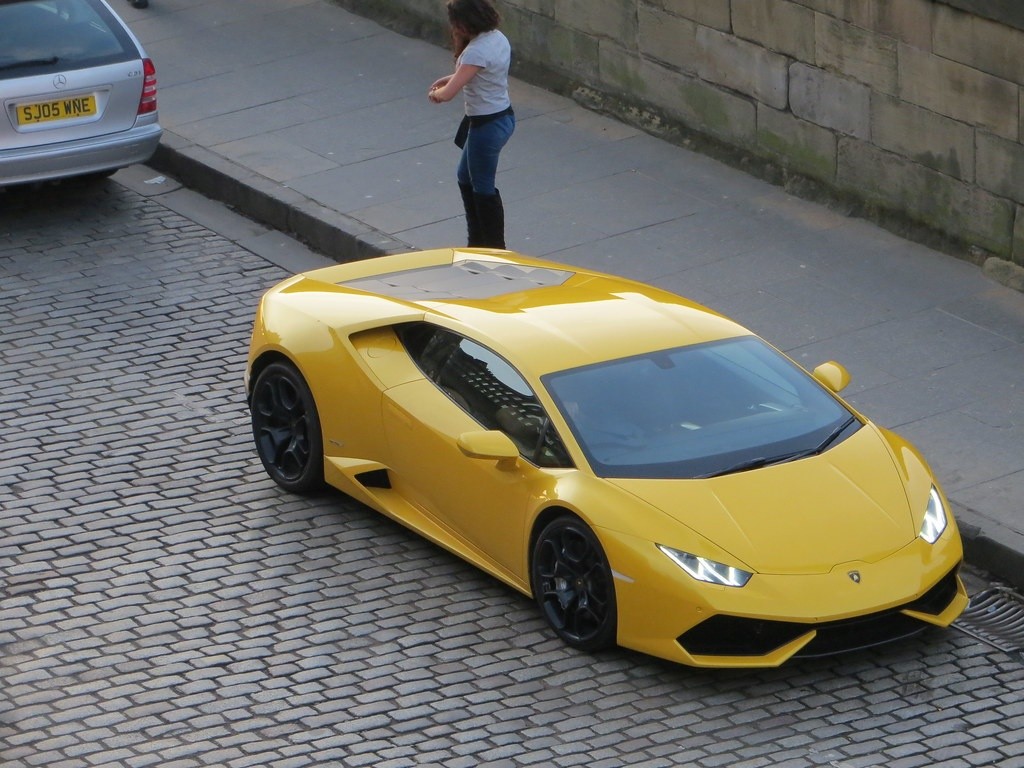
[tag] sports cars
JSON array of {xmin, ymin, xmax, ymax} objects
[{"xmin": 243, "ymin": 248, "xmax": 969, "ymax": 668}]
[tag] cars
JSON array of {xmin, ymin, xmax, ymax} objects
[{"xmin": 0, "ymin": 0, "xmax": 163, "ymax": 185}]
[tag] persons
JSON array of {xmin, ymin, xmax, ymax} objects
[{"xmin": 428, "ymin": 0, "xmax": 520, "ymax": 250}]
